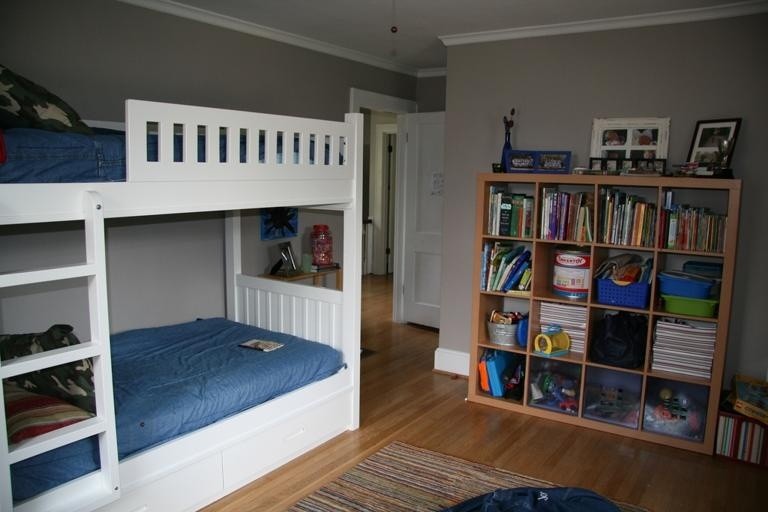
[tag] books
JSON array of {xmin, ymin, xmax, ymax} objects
[
  {"xmin": 487, "ymin": 183, "xmax": 728, "ymax": 253},
  {"xmin": 476, "ymin": 240, "xmax": 533, "ymax": 294},
  {"xmin": 237, "ymin": 339, "xmax": 285, "ymax": 353},
  {"xmin": 649, "ymin": 318, "xmax": 718, "ymax": 381},
  {"xmin": 537, "ymin": 302, "xmax": 588, "ymax": 354},
  {"xmin": 713, "ymin": 415, "xmax": 764, "ymax": 465}
]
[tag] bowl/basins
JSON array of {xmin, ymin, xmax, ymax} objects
[{"xmin": 487, "ymin": 320, "xmax": 520, "ymax": 347}]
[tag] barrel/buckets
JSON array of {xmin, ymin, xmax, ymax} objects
[
  {"xmin": 552, "ymin": 247, "xmax": 593, "ymax": 300},
  {"xmin": 487, "ymin": 319, "xmax": 524, "ymax": 347}
]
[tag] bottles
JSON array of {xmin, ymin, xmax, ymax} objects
[{"xmin": 310, "ymin": 223, "xmax": 333, "ymax": 267}]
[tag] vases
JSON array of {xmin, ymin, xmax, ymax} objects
[{"xmin": 500, "ymin": 132, "xmax": 513, "ymax": 172}]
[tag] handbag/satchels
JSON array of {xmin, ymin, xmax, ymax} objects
[{"xmin": 590, "ymin": 311, "xmax": 648, "ymax": 369}]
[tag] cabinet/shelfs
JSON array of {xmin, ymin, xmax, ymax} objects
[
  {"xmin": 257, "ymin": 267, "xmax": 343, "ymax": 291},
  {"xmin": 466, "ymin": 172, "xmax": 744, "ymax": 459}
]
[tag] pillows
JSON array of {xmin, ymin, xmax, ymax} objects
[
  {"xmin": 0, "ymin": 323, "xmax": 119, "ymax": 444},
  {"xmin": 0, "ymin": 65, "xmax": 94, "ymax": 134}
]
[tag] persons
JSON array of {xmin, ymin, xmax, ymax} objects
[{"xmin": 601, "ymin": 127, "xmax": 657, "ymax": 170}]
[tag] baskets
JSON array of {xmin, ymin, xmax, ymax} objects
[{"xmin": 596, "ymin": 279, "xmax": 652, "ymax": 310}]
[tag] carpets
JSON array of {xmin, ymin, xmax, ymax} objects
[{"xmin": 281, "ymin": 440, "xmax": 649, "ymax": 511}]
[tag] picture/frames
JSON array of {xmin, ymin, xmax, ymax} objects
[
  {"xmin": 270, "ymin": 241, "xmax": 299, "ymax": 275},
  {"xmin": 685, "ymin": 115, "xmax": 743, "ymax": 172},
  {"xmin": 587, "ymin": 115, "xmax": 671, "ymax": 175}
]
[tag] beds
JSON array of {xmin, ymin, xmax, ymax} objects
[{"xmin": 0, "ymin": 97, "xmax": 366, "ymax": 511}]
